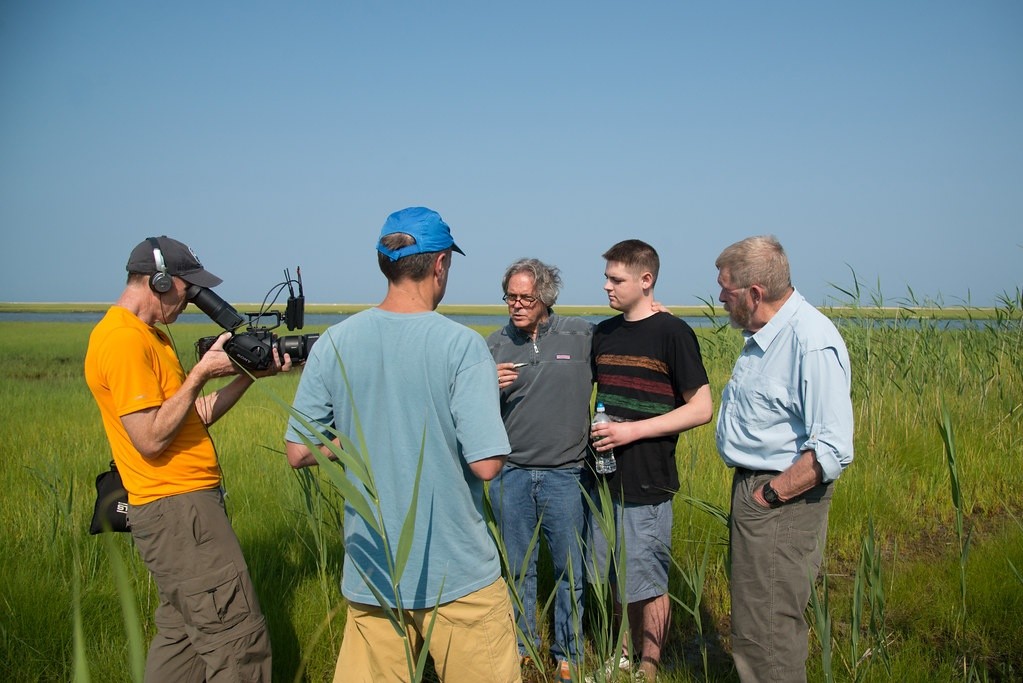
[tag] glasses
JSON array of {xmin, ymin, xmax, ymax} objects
[{"xmin": 502, "ymin": 293, "xmax": 541, "ymax": 307}]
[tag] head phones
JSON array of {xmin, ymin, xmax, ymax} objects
[{"xmin": 146, "ymin": 237, "xmax": 173, "ymax": 294}]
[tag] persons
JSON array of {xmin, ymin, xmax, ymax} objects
[
  {"xmin": 84, "ymin": 236, "xmax": 293, "ymax": 683},
  {"xmin": 715, "ymin": 235, "xmax": 854, "ymax": 683},
  {"xmin": 486, "ymin": 257, "xmax": 674, "ymax": 683},
  {"xmin": 580, "ymin": 239, "xmax": 713, "ymax": 683},
  {"xmin": 285, "ymin": 206, "xmax": 522, "ymax": 683}
]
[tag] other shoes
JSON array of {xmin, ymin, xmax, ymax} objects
[
  {"xmin": 518, "ymin": 655, "xmax": 531, "ymax": 665},
  {"xmin": 557, "ymin": 659, "xmax": 581, "ymax": 683},
  {"xmin": 585, "ymin": 651, "xmax": 634, "ymax": 683}
]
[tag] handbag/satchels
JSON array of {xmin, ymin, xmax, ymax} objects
[{"xmin": 89, "ymin": 461, "xmax": 132, "ymax": 534}]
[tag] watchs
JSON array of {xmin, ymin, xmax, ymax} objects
[{"xmin": 763, "ymin": 481, "xmax": 785, "ymax": 509}]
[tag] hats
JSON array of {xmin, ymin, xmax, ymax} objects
[
  {"xmin": 126, "ymin": 235, "xmax": 224, "ymax": 287},
  {"xmin": 376, "ymin": 206, "xmax": 466, "ymax": 262}
]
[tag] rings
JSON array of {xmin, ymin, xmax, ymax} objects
[{"xmin": 498, "ymin": 377, "xmax": 501, "ymax": 383}]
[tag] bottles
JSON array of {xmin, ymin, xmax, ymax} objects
[{"xmin": 592, "ymin": 402, "xmax": 616, "ymax": 473}]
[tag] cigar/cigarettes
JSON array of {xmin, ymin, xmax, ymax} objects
[{"xmin": 512, "ymin": 362, "xmax": 528, "ymax": 368}]
